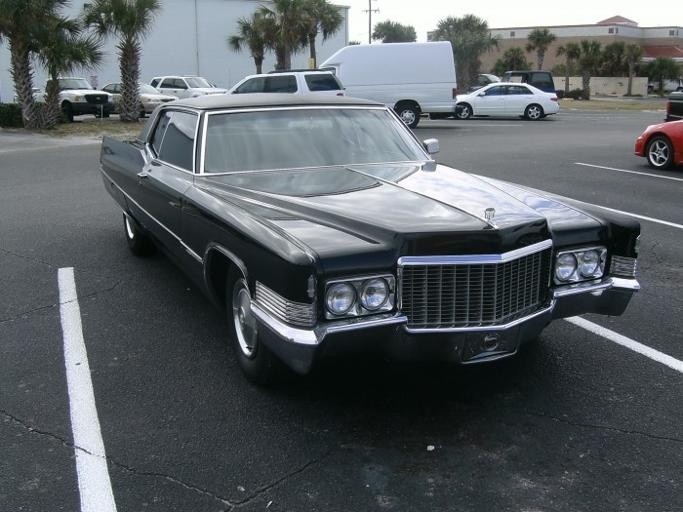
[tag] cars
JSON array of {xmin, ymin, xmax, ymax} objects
[
  {"xmin": 665, "ymin": 85, "xmax": 683, "ymax": 122},
  {"xmin": 634, "ymin": 119, "xmax": 683, "ymax": 169},
  {"xmin": 98, "ymin": 94, "xmax": 642, "ymax": 383}
]
